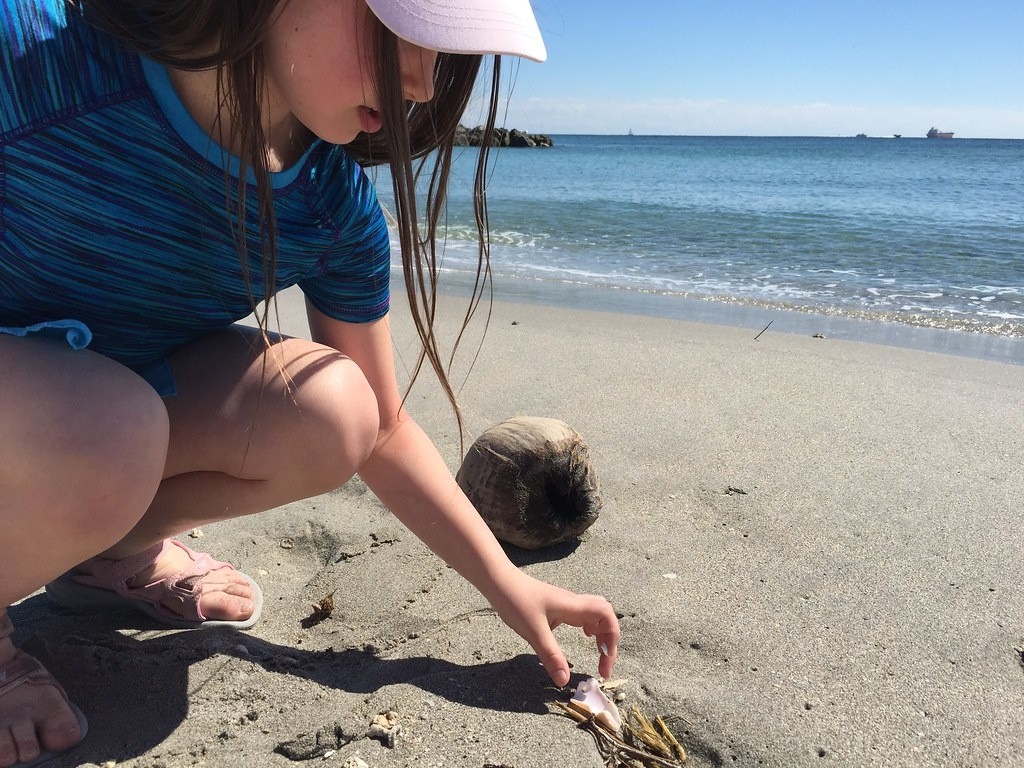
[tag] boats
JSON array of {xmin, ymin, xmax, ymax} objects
[{"xmin": 926, "ymin": 127, "xmax": 954, "ymax": 138}]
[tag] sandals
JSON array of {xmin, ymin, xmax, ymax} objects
[
  {"xmin": 45, "ymin": 538, "xmax": 263, "ymax": 630},
  {"xmin": 0, "ymin": 614, "xmax": 88, "ymax": 768}
]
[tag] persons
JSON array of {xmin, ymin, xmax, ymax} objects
[{"xmin": 1, "ymin": 1, "xmax": 619, "ymax": 764}]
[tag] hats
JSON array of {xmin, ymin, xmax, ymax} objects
[{"xmin": 366, "ymin": 0, "xmax": 547, "ymax": 63}]
